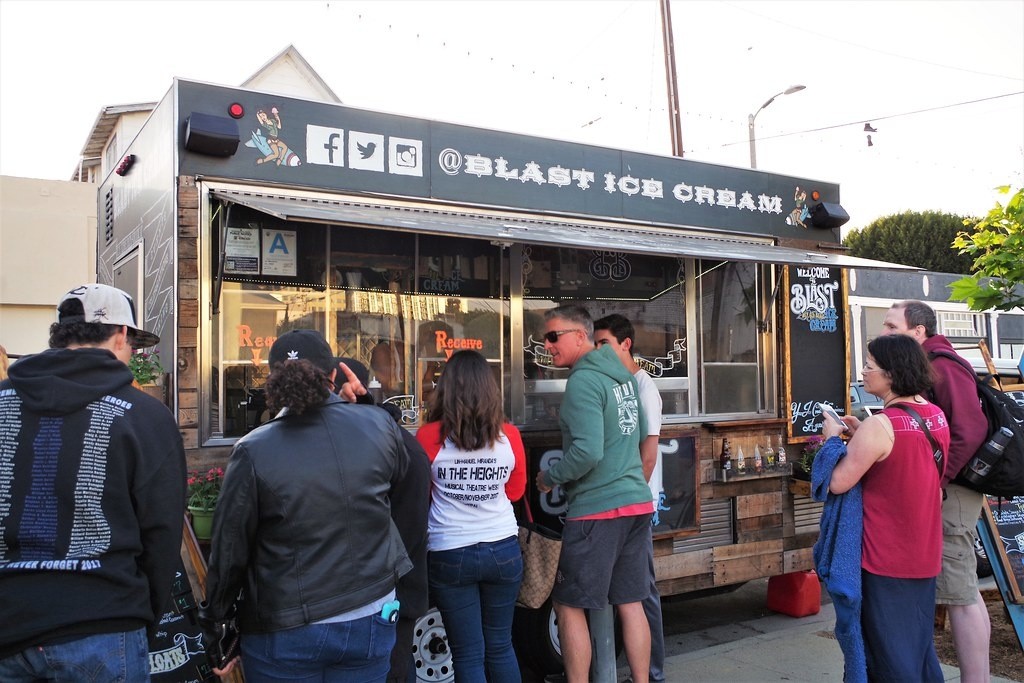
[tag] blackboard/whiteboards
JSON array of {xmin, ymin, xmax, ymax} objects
[
  {"xmin": 982, "ymin": 384, "xmax": 1024, "ymax": 605},
  {"xmin": 782, "ymin": 264, "xmax": 854, "ymax": 441}
]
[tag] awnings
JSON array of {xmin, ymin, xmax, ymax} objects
[{"xmin": 207, "ymin": 192, "xmax": 926, "ymax": 328}]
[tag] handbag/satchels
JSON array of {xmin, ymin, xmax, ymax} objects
[{"xmin": 516, "ymin": 517, "xmax": 563, "ymax": 609}]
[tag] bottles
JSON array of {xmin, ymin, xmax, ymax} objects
[
  {"xmin": 752, "ymin": 447, "xmax": 762, "ymax": 475},
  {"xmin": 777, "ymin": 447, "xmax": 786, "ymax": 467},
  {"xmin": 766, "ymin": 448, "xmax": 775, "ymax": 473},
  {"xmin": 368, "ymin": 377, "xmax": 383, "ymax": 407},
  {"xmin": 965, "ymin": 427, "xmax": 1014, "ymax": 485},
  {"xmin": 735, "ymin": 444, "xmax": 746, "ymax": 475},
  {"xmin": 720, "ymin": 438, "xmax": 731, "ymax": 470}
]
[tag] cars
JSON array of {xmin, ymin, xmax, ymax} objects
[{"xmin": 849, "ymin": 357, "xmax": 1024, "ymax": 579}]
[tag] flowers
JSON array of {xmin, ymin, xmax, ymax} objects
[
  {"xmin": 800, "ymin": 436, "xmax": 823, "ymax": 472},
  {"xmin": 186, "ymin": 468, "xmax": 223, "ymax": 507},
  {"xmin": 129, "ymin": 349, "xmax": 162, "ymax": 382}
]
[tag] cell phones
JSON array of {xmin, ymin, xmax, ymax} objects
[{"xmin": 818, "ymin": 403, "xmax": 849, "ymax": 430}]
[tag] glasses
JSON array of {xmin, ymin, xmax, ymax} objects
[{"xmin": 542, "ymin": 327, "xmax": 588, "ymax": 343}]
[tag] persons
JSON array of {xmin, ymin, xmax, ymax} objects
[
  {"xmin": 883, "ymin": 299, "xmax": 992, "ymax": 683},
  {"xmin": 536, "ymin": 305, "xmax": 655, "ymax": 683},
  {"xmin": 416, "ymin": 348, "xmax": 527, "ymax": 683},
  {"xmin": 594, "ymin": 314, "xmax": 665, "ymax": 683},
  {"xmin": 0, "ymin": 282, "xmax": 186, "ymax": 683},
  {"xmin": 333, "ymin": 360, "xmax": 431, "ymax": 682},
  {"xmin": 203, "ymin": 328, "xmax": 411, "ymax": 683},
  {"xmin": 371, "ymin": 338, "xmax": 424, "ymax": 397},
  {"xmin": 822, "ymin": 334, "xmax": 950, "ymax": 683}
]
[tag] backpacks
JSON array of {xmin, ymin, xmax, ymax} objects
[{"xmin": 928, "ymin": 351, "xmax": 1024, "ymax": 501}]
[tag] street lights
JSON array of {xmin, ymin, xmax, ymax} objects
[{"xmin": 748, "ymin": 84, "xmax": 808, "ymax": 169}]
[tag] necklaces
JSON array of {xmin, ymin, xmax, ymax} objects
[{"xmin": 884, "ymin": 395, "xmax": 923, "ymax": 408}]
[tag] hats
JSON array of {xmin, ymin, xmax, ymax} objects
[
  {"xmin": 334, "ymin": 358, "xmax": 369, "ymax": 392},
  {"xmin": 56, "ymin": 283, "xmax": 160, "ymax": 349},
  {"xmin": 269, "ymin": 329, "xmax": 334, "ymax": 378}
]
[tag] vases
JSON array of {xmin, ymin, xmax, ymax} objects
[
  {"xmin": 188, "ymin": 504, "xmax": 216, "ymax": 539},
  {"xmin": 794, "ymin": 461, "xmax": 811, "ymax": 481},
  {"xmin": 141, "ymin": 383, "xmax": 164, "ymax": 404}
]
